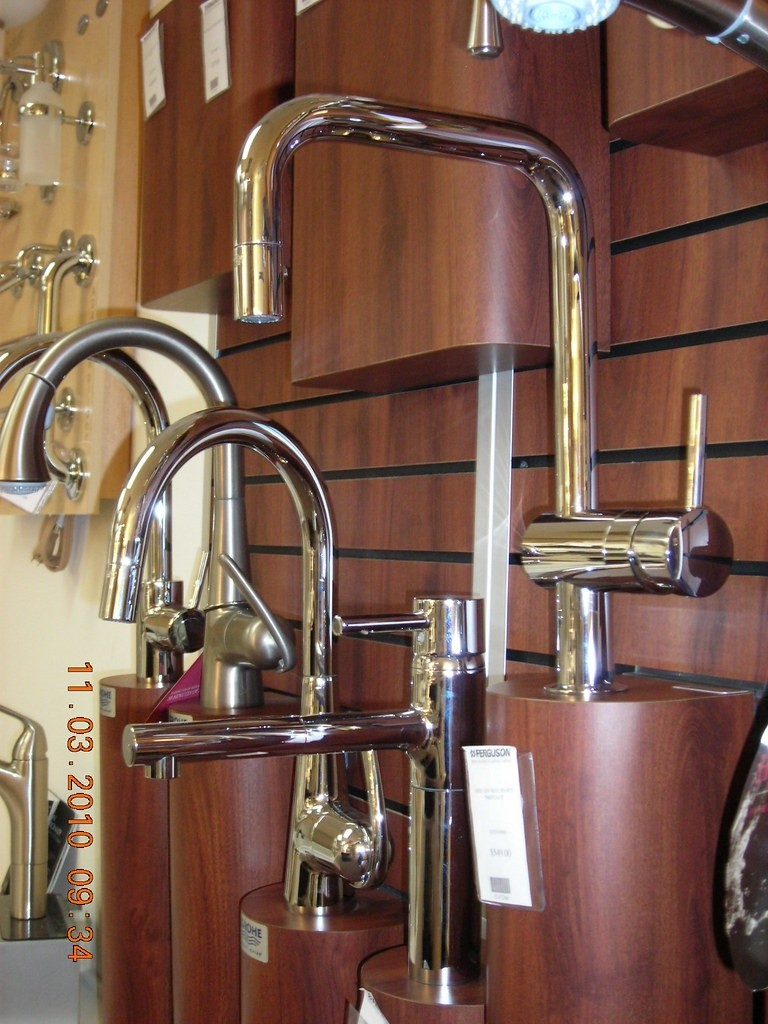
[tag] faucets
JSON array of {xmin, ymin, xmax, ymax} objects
[
  {"xmin": 466, "ymin": 0, "xmax": 768, "ymax": 73},
  {"xmin": 123, "ymin": 597, "xmax": 487, "ymax": 986},
  {"xmin": 99, "ymin": 409, "xmax": 392, "ymax": 918},
  {"xmin": 0, "ymin": 705, "xmax": 48, "ymax": 920},
  {"xmin": 233, "ymin": 94, "xmax": 734, "ymax": 695},
  {"xmin": 0, "ymin": 333, "xmax": 205, "ymax": 682},
  {"xmin": 0, "ymin": 317, "xmax": 297, "ymax": 708}
]
